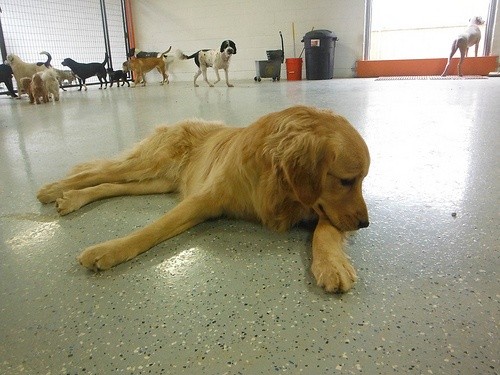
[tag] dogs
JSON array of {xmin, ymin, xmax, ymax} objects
[
  {"xmin": 0, "ymin": 63, "xmax": 18, "ymax": 97},
  {"xmin": 61, "ymin": 52, "xmax": 108, "ymax": 91},
  {"xmin": 36, "ymin": 103, "xmax": 369, "ymax": 296},
  {"xmin": 122, "ymin": 46, "xmax": 174, "ymax": 88},
  {"xmin": 106, "ymin": 68, "xmax": 130, "ymax": 87},
  {"xmin": 4, "ymin": 51, "xmax": 77, "ymax": 104},
  {"xmin": 175, "ymin": 40, "xmax": 236, "ymax": 87},
  {"xmin": 441, "ymin": 15, "xmax": 485, "ymax": 76}
]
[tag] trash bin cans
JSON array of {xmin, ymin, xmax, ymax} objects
[{"xmin": 301, "ymin": 29, "xmax": 337, "ymax": 80}]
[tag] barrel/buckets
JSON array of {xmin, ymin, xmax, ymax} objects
[{"xmin": 285, "ymin": 57, "xmax": 303, "ymax": 81}]
